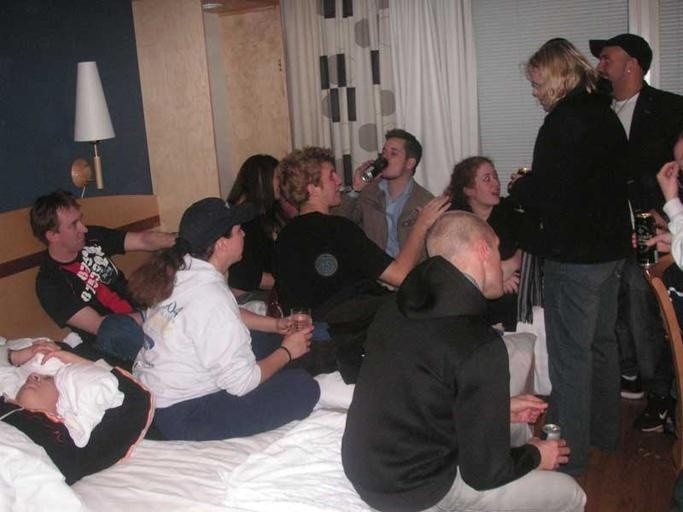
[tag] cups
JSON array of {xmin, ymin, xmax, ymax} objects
[{"xmin": 289, "ymin": 304, "xmax": 312, "ymax": 335}]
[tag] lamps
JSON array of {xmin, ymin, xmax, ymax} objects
[{"xmin": 71, "ymin": 60, "xmax": 116, "ymax": 190}]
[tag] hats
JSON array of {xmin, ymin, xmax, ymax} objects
[
  {"xmin": 590, "ymin": 34, "xmax": 652, "ymax": 74},
  {"xmin": 178, "ymin": 198, "xmax": 256, "ymax": 258}
]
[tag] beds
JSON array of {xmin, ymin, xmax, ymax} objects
[{"xmin": 0, "ymin": 370, "xmax": 371, "ymax": 511}]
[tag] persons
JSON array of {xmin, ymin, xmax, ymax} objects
[
  {"xmin": 30, "ymin": 189, "xmax": 182, "ymax": 361},
  {"xmin": 510, "ymin": 35, "xmax": 683, "ymax": 477},
  {"xmin": 341, "ymin": 211, "xmax": 587, "ymax": 512},
  {"xmin": 126, "ymin": 197, "xmax": 321, "ymax": 441},
  {"xmin": 226, "ymin": 129, "xmax": 524, "ymax": 374},
  {"xmin": 0, "ymin": 339, "xmax": 156, "ymax": 487}
]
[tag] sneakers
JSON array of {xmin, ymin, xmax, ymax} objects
[
  {"xmin": 621, "ymin": 375, "xmax": 644, "ymax": 400},
  {"xmin": 634, "ymin": 390, "xmax": 676, "ymax": 432}
]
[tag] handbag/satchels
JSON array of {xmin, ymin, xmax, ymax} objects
[{"xmin": 515, "ymin": 305, "xmax": 552, "ymax": 396}]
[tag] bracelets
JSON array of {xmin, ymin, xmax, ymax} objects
[{"xmin": 7, "ymin": 349, "xmax": 21, "ymax": 368}]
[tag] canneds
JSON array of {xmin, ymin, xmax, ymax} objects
[
  {"xmin": 541, "ymin": 424, "xmax": 561, "ymax": 441},
  {"xmin": 361, "ymin": 156, "xmax": 387, "ymax": 183},
  {"xmin": 635, "ymin": 213, "xmax": 655, "ymax": 268},
  {"xmin": 511, "ymin": 167, "xmax": 532, "ymax": 214}
]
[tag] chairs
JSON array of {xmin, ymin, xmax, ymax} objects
[{"xmin": 643, "ymin": 267, "xmax": 683, "ymax": 471}]
